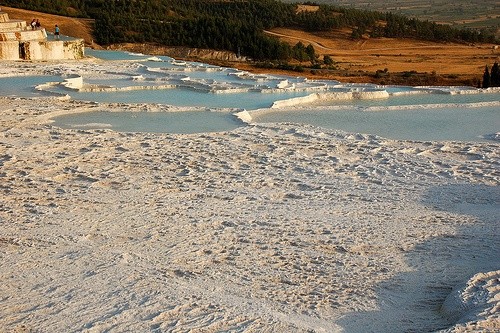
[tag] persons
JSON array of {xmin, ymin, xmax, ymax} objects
[
  {"xmin": 20, "ymin": 43, "xmax": 26, "ymax": 60},
  {"xmin": 30, "ymin": 19, "xmax": 36, "ymax": 31},
  {"xmin": 35, "ymin": 19, "xmax": 41, "ymax": 30},
  {"xmin": 53, "ymin": 24, "xmax": 60, "ymax": 40}
]
